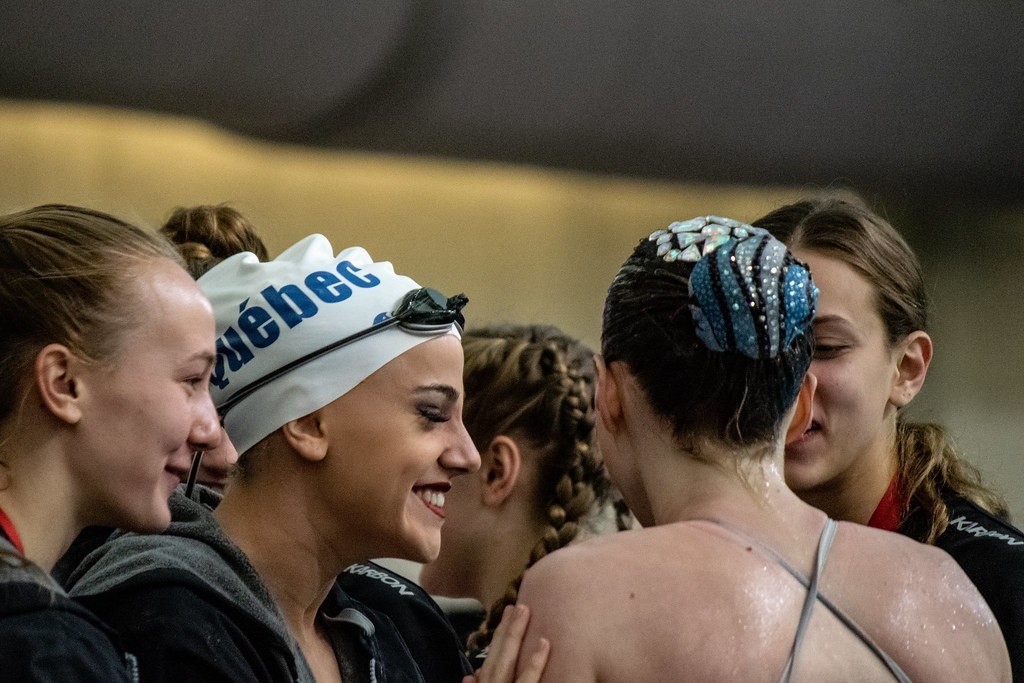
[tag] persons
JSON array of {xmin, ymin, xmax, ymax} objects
[
  {"xmin": 49, "ymin": 234, "xmax": 475, "ymax": 683},
  {"xmin": 413, "ymin": 325, "xmax": 637, "ymax": 680},
  {"xmin": 512, "ymin": 217, "xmax": 1011, "ymax": 682},
  {"xmin": 0, "ymin": 204, "xmax": 217, "ymax": 682},
  {"xmin": 740, "ymin": 202, "xmax": 1024, "ymax": 682},
  {"xmin": 139, "ymin": 207, "xmax": 278, "ymax": 500}
]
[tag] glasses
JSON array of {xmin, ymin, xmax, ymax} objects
[{"xmin": 215, "ymin": 286, "xmax": 468, "ymax": 423}]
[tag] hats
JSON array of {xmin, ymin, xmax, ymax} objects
[{"xmin": 196, "ymin": 233, "xmax": 461, "ymax": 459}]
